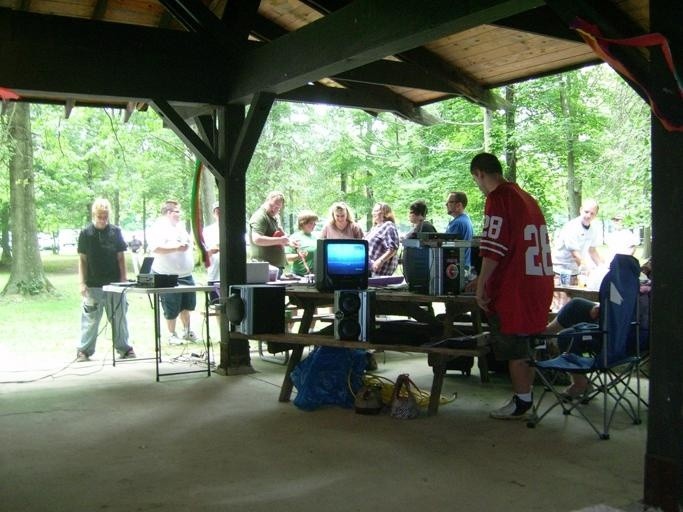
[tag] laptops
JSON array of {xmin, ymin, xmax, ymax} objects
[{"xmin": 139, "ymin": 257, "xmax": 155, "ymax": 274}]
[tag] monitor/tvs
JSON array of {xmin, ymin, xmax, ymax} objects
[
  {"xmin": 401, "ymin": 231, "xmax": 462, "ymax": 293},
  {"xmin": 316, "ymin": 239, "xmax": 370, "ymax": 295}
]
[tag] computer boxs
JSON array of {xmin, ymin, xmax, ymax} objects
[{"xmin": 136, "ymin": 274, "xmax": 179, "ymax": 288}]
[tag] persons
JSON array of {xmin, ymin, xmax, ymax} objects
[
  {"xmin": 445, "ymin": 192, "xmax": 474, "ymax": 266},
  {"xmin": 128, "ymin": 232, "xmax": 143, "ymax": 281},
  {"xmin": 201, "ymin": 201, "xmax": 220, "ymax": 325},
  {"xmin": 322, "ymin": 203, "xmax": 364, "ymax": 239},
  {"xmin": 283, "ymin": 210, "xmax": 321, "ymax": 332},
  {"xmin": 549, "ymin": 197, "xmax": 638, "ymax": 312},
  {"xmin": 366, "ymin": 202, "xmax": 399, "ymax": 275},
  {"xmin": 75, "ymin": 199, "xmax": 137, "ymax": 362},
  {"xmin": 147, "ymin": 199, "xmax": 200, "ymax": 345},
  {"xmin": 466, "ymin": 152, "xmax": 555, "ymax": 421},
  {"xmin": 249, "ymin": 189, "xmax": 301, "ymax": 279},
  {"xmin": 407, "ymin": 201, "xmax": 436, "ymax": 236}
]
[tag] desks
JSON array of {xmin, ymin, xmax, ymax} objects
[
  {"xmin": 279, "ymin": 285, "xmax": 477, "ymax": 417},
  {"xmin": 102, "ymin": 282, "xmax": 216, "ymax": 383},
  {"xmin": 208, "ymin": 276, "xmax": 404, "ymax": 365}
]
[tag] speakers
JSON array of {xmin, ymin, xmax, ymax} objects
[
  {"xmin": 334, "ymin": 289, "xmax": 377, "ymax": 342},
  {"xmin": 228, "ymin": 284, "xmax": 286, "ymax": 336}
]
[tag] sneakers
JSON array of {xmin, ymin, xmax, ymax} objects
[
  {"xmin": 169, "ymin": 334, "xmax": 182, "ymax": 345},
  {"xmin": 563, "ymin": 380, "xmax": 592, "ymax": 397},
  {"xmin": 183, "ymin": 331, "xmax": 201, "ymax": 341},
  {"xmin": 490, "ymin": 395, "xmax": 536, "ymax": 420}
]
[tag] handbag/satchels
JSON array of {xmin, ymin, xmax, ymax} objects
[
  {"xmin": 355, "ymin": 386, "xmax": 383, "ymax": 415},
  {"xmin": 390, "ymin": 397, "xmax": 419, "ymax": 420}
]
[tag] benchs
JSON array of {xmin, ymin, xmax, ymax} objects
[{"xmin": 251, "ymin": 334, "xmax": 490, "ymax": 417}]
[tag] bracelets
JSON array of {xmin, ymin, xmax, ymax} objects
[
  {"xmin": 176, "ymin": 246, "xmax": 179, "ymax": 253},
  {"xmin": 381, "ymin": 259, "xmax": 385, "ymax": 263}
]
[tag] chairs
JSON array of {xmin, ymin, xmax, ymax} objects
[{"xmin": 525, "ymin": 254, "xmax": 640, "ymax": 441}]
